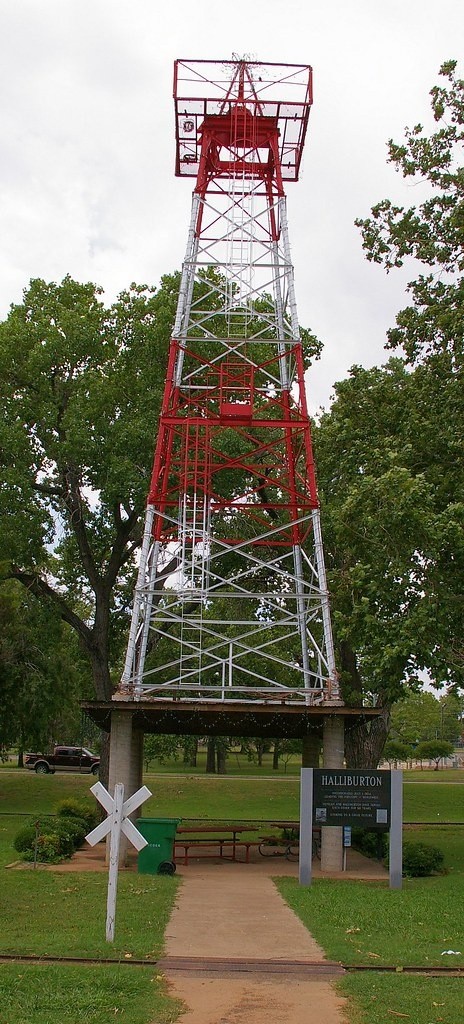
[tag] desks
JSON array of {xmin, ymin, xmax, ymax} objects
[
  {"xmin": 271, "ymin": 824, "xmax": 321, "ymax": 856},
  {"xmin": 176, "ymin": 826, "xmax": 259, "ymax": 863}
]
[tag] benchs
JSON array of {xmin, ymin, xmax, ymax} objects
[
  {"xmin": 174, "ymin": 838, "xmax": 240, "ymax": 858},
  {"xmin": 173, "ymin": 842, "xmax": 261, "ymax": 866},
  {"xmin": 258, "ymin": 836, "xmax": 321, "ymax": 862}
]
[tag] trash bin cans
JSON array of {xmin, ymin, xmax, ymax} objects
[
  {"xmin": 135, "ymin": 817, "xmax": 182, "ymax": 875},
  {"xmin": 453, "ymin": 762, "xmax": 458, "ymax": 768}
]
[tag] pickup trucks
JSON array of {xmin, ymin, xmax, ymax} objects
[{"xmin": 23, "ymin": 747, "xmax": 103, "ymax": 776}]
